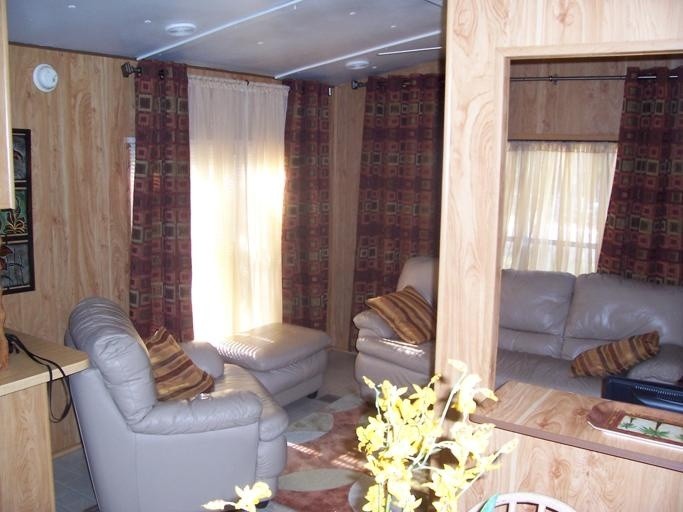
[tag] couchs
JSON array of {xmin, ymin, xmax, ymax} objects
[{"xmin": 353, "ymin": 258, "xmax": 682, "ymax": 407}]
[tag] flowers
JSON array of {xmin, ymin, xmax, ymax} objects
[{"xmin": 201, "ymin": 477, "xmax": 275, "ymax": 511}]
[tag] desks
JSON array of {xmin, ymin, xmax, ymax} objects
[
  {"xmin": 1, "ymin": 327, "xmax": 91, "ymax": 512},
  {"xmin": 458, "ymin": 378, "xmax": 682, "ymax": 512}
]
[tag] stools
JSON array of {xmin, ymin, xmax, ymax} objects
[{"xmin": 216, "ymin": 323, "xmax": 333, "ymax": 404}]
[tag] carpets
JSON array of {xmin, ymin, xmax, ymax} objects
[{"xmin": 84, "ymin": 390, "xmax": 384, "ymax": 512}]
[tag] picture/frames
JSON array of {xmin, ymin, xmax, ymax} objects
[{"xmin": 0, "ymin": 129, "xmax": 37, "ymax": 296}]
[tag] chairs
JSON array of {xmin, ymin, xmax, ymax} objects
[
  {"xmin": 64, "ymin": 296, "xmax": 290, "ymax": 512},
  {"xmin": 466, "ymin": 490, "xmax": 577, "ymax": 512}
]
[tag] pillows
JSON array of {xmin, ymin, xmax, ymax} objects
[
  {"xmin": 569, "ymin": 331, "xmax": 661, "ymax": 381},
  {"xmin": 363, "ymin": 286, "xmax": 436, "ymax": 346},
  {"xmin": 142, "ymin": 325, "xmax": 213, "ymax": 403}
]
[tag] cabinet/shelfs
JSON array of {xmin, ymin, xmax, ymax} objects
[{"xmin": 1, "ymin": 1, "xmax": 19, "ymax": 212}]
